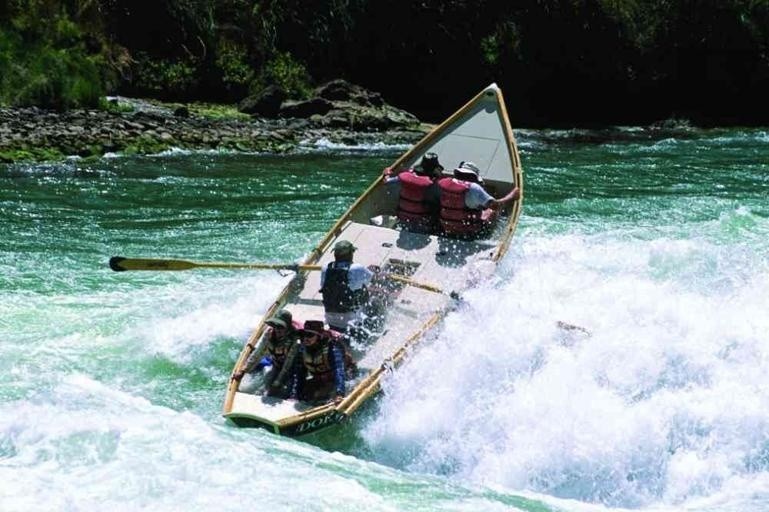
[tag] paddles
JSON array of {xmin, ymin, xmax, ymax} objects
[{"xmin": 110, "ymin": 256, "xmax": 321, "ymax": 272}]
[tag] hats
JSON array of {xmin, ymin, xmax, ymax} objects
[
  {"xmin": 413, "ymin": 152, "xmax": 444, "ymax": 175},
  {"xmin": 333, "ymin": 239, "xmax": 358, "ymax": 254},
  {"xmin": 453, "ymin": 162, "xmax": 485, "ymax": 189},
  {"xmin": 298, "ymin": 320, "xmax": 326, "ymax": 340},
  {"xmin": 263, "ymin": 309, "xmax": 293, "ymax": 331}
]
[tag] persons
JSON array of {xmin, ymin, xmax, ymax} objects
[
  {"xmin": 437, "ymin": 160, "xmax": 520, "ymax": 242},
  {"xmin": 382, "ymin": 153, "xmax": 445, "ymax": 236},
  {"xmin": 318, "ymin": 240, "xmax": 390, "ymax": 342},
  {"xmin": 266, "ymin": 319, "xmax": 359, "ymax": 406},
  {"xmin": 231, "ymin": 309, "xmax": 304, "ymax": 396}
]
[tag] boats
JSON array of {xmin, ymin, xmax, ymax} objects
[{"xmin": 222, "ymin": 78, "xmax": 522, "ymax": 437}]
